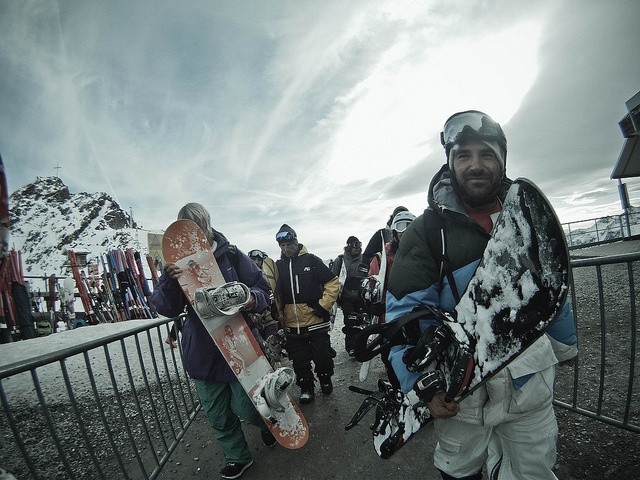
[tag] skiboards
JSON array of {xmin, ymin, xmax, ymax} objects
[{"xmin": 82, "ymin": 270, "xmax": 108, "ymax": 324}]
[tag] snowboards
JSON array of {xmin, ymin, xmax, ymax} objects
[
  {"xmin": 161, "ymin": 219, "xmax": 310, "ymax": 451},
  {"xmin": 49, "ymin": 274, "xmax": 55, "ymax": 310},
  {"xmin": 371, "ymin": 178, "xmax": 573, "ymax": 459},
  {"xmin": 64, "ymin": 278, "xmax": 76, "ymax": 330},
  {"xmin": 360, "ymin": 233, "xmax": 387, "ymax": 382},
  {"xmin": 329, "ymin": 259, "xmax": 347, "ymax": 331},
  {"xmin": 261, "ymin": 256, "xmax": 285, "ymax": 326}
]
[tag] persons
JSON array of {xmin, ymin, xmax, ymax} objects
[
  {"xmin": 248, "ymin": 249, "xmax": 283, "ymax": 333},
  {"xmin": 150, "ymin": 203, "xmax": 278, "ymax": 479},
  {"xmin": 363, "ymin": 206, "xmax": 409, "ymax": 269},
  {"xmin": 275, "ymin": 223, "xmax": 341, "ymax": 403},
  {"xmin": 331, "ymin": 236, "xmax": 368, "ymax": 359},
  {"xmin": 361, "ymin": 211, "xmax": 417, "ymax": 390},
  {"xmin": 379, "ymin": 109, "xmax": 580, "ymax": 480},
  {"xmin": 165, "ymin": 312, "xmax": 186, "ymax": 348}
]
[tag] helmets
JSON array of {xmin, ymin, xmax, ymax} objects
[{"xmin": 390, "ymin": 211, "xmax": 416, "ymax": 231}]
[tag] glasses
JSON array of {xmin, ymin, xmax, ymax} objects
[
  {"xmin": 352, "ymin": 242, "xmax": 362, "ymax": 248},
  {"xmin": 392, "ymin": 221, "xmax": 412, "ymax": 232},
  {"xmin": 441, "ymin": 111, "xmax": 507, "ymax": 150},
  {"xmin": 277, "ymin": 232, "xmax": 294, "ymax": 242},
  {"xmin": 249, "ymin": 250, "xmax": 264, "ymax": 259}
]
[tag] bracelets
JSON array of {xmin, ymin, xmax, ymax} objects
[{"xmin": 413, "ymin": 370, "xmax": 447, "ymax": 401}]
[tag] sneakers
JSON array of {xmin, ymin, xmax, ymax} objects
[
  {"xmin": 221, "ymin": 460, "xmax": 254, "ymax": 479},
  {"xmin": 349, "ymin": 349, "xmax": 355, "ymax": 357},
  {"xmin": 300, "ymin": 382, "xmax": 315, "ymax": 404},
  {"xmin": 262, "ymin": 430, "xmax": 277, "ymax": 447},
  {"xmin": 319, "ymin": 373, "xmax": 332, "ymax": 394}
]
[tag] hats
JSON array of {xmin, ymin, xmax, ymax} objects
[{"xmin": 278, "ymin": 224, "xmax": 297, "ymax": 243}]
